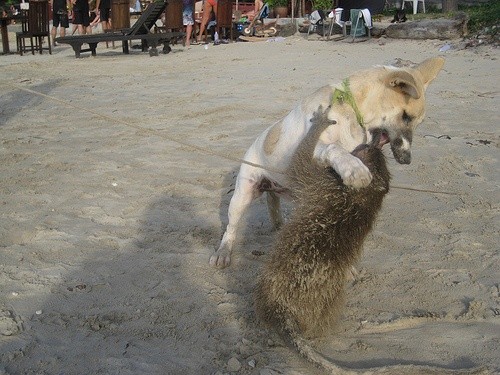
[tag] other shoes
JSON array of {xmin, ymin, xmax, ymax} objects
[
  {"xmin": 215, "ymin": 39, "xmax": 221, "ymax": 44},
  {"xmin": 185, "ymin": 42, "xmax": 191, "ymax": 49},
  {"xmin": 196, "ymin": 36, "xmax": 202, "ymax": 46},
  {"xmin": 220, "ymin": 38, "xmax": 229, "ymax": 44}
]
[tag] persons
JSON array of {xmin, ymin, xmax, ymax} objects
[
  {"xmin": 195, "ymin": 0, "xmax": 220, "ymax": 45},
  {"xmin": 95, "ymin": 0, "xmax": 112, "ymax": 31},
  {"xmin": 71, "ymin": 0, "xmax": 92, "ymax": 35},
  {"xmin": 51, "ymin": 0, "xmax": 69, "ymax": 47},
  {"xmin": 182, "ymin": 0, "xmax": 194, "ymax": 49}
]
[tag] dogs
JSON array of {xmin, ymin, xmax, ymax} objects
[{"xmin": 210, "ymin": 56, "xmax": 443, "ymax": 271}]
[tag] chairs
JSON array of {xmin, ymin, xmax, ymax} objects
[
  {"xmin": 16, "ymin": 1, "xmax": 51, "ymax": 56},
  {"xmin": 54, "ymin": 1, "xmax": 184, "ymax": 59},
  {"xmin": 205, "ymin": 0, "xmax": 233, "ymax": 44}
]
